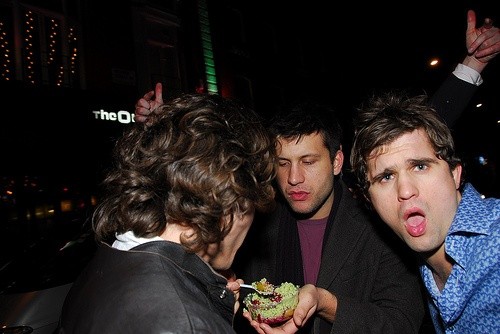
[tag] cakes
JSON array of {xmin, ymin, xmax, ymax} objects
[{"xmin": 243, "ymin": 278, "xmax": 298, "ymax": 323}]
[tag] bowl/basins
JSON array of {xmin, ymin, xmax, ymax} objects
[{"xmin": 243, "ymin": 286, "xmax": 301, "ymax": 325}]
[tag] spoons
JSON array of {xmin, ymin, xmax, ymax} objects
[{"xmin": 240, "ymin": 282, "xmax": 274, "ymax": 295}]
